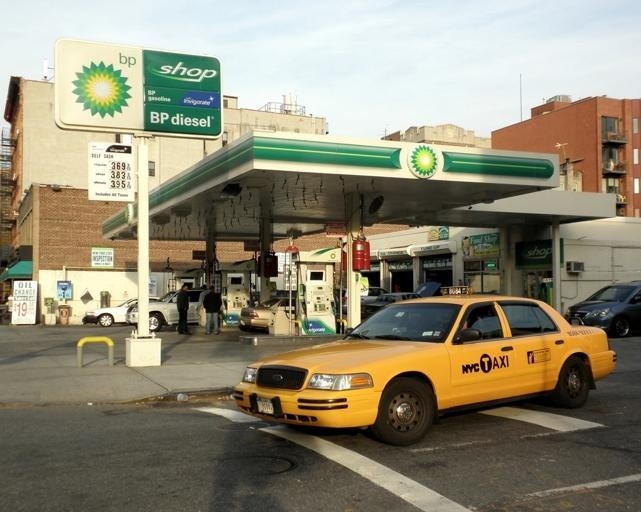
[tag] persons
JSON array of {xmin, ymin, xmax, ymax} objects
[
  {"xmin": 177, "ymin": 284, "xmax": 192, "ymax": 335},
  {"xmin": 203, "ymin": 285, "xmax": 223, "ymax": 335},
  {"xmin": 197, "ymin": 284, "xmax": 211, "ymax": 333}
]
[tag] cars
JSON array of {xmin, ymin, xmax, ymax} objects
[
  {"xmin": 564, "ymin": 281, "xmax": 641, "ymax": 338},
  {"xmin": 337, "ymin": 281, "xmax": 442, "ymax": 316},
  {"xmin": 234, "ymin": 294, "xmax": 615, "ymax": 446},
  {"xmin": 238, "ymin": 296, "xmax": 298, "ymax": 332},
  {"xmin": 85, "ymin": 296, "xmax": 162, "ymax": 326}
]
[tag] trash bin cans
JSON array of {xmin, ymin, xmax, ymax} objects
[{"xmin": 58, "ymin": 305, "xmax": 70, "ymax": 326}]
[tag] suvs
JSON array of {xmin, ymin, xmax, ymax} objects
[{"xmin": 127, "ymin": 290, "xmax": 207, "ymax": 330}]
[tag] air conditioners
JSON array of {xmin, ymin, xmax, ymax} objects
[{"xmin": 567, "ymin": 261, "xmax": 584, "ymax": 272}]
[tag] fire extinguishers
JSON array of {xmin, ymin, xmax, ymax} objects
[
  {"xmin": 351, "ymin": 234, "xmax": 370, "ymax": 270},
  {"xmin": 342, "ymin": 251, "xmax": 347, "ymax": 271}
]
